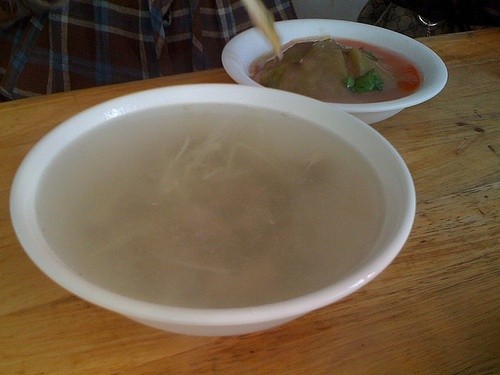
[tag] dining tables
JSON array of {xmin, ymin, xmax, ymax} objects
[{"xmin": 1, "ymin": 21, "xmax": 499, "ymax": 375}]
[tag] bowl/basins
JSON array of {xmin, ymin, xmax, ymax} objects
[
  {"xmin": 224, "ymin": 16, "xmax": 450, "ymax": 129},
  {"xmin": 7, "ymin": 83, "xmax": 419, "ymax": 340}
]
